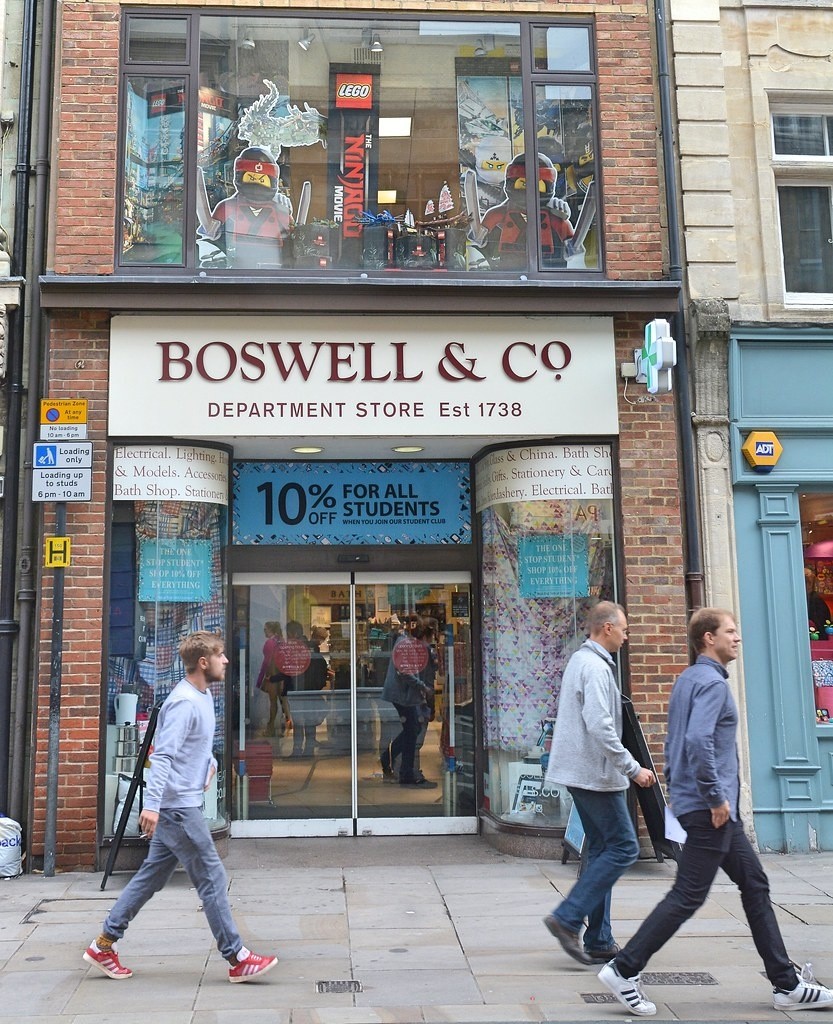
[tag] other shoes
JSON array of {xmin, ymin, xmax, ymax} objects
[
  {"xmin": 400, "ymin": 777, "xmax": 437, "ymax": 790},
  {"xmin": 262, "ymin": 725, "xmax": 277, "ymax": 737},
  {"xmin": 382, "ymin": 772, "xmax": 400, "ymax": 784},
  {"xmin": 279, "ymin": 721, "xmax": 293, "ymax": 738},
  {"xmin": 283, "ymin": 750, "xmax": 316, "ymax": 761}
]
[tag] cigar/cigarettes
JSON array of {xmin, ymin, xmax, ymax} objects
[{"xmin": 140, "ymin": 834, "xmax": 148, "ymax": 840}]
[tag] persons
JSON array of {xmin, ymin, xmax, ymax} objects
[
  {"xmin": 81, "ymin": 630, "xmax": 278, "ymax": 984},
  {"xmin": 543, "ymin": 602, "xmax": 657, "ymax": 966},
  {"xmin": 253, "ymin": 611, "xmax": 440, "ymax": 789},
  {"xmin": 596, "ymin": 608, "xmax": 833, "ymax": 1017}
]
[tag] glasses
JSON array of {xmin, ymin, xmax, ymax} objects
[{"xmin": 601, "ymin": 622, "xmax": 627, "ymax": 635}]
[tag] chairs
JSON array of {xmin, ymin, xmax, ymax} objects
[
  {"xmin": 237, "ymin": 27, "xmax": 256, "ymax": 49},
  {"xmin": 298, "ymin": 25, "xmax": 316, "ymax": 51}
]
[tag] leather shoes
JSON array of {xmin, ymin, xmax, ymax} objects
[
  {"xmin": 582, "ymin": 942, "xmax": 623, "ymax": 964},
  {"xmin": 542, "ymin": 915, "xmax": 594, "ymax": 965}
]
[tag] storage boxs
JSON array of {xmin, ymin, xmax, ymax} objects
[{"xmin": 291, "ymin": 224, "xmax": 466, "ymax": 272}]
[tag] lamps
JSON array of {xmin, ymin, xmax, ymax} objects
[
  {"xmin": 361, "ymin": 27, "xmax": 384, "ymax": 51},
  {"xmin": 473, "ymin": 34, "xmax": 494, "ymax": 56}
]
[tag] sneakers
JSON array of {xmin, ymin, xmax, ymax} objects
[
  {"xmin": 228, "ymin": 946, "xmax": 279, "ymax": 983},
  {"xmin": 772, "ymin": 963, "xmax": 833, "ymax": 1011},
  {"xmin": 597, "ymin": 958, "xmax": 656, "ymax": 1016},
  {"xmin": 83, "ymin": 940, "xmax": 132, "ymax": 979}
]
